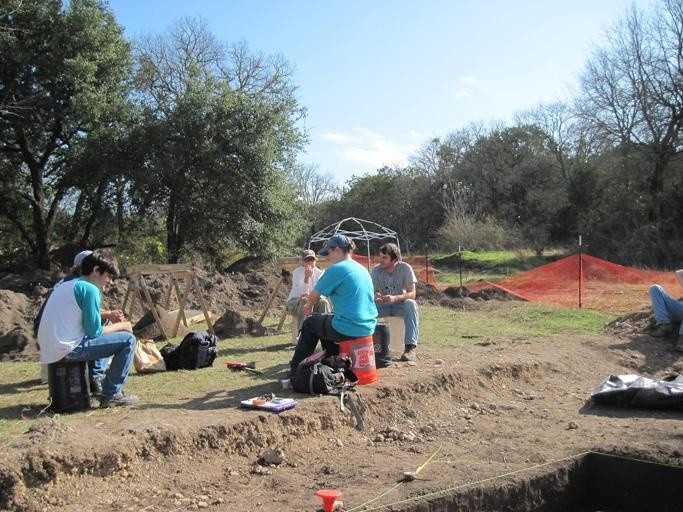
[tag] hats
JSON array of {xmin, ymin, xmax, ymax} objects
[
  {"xmin": 73, "ymin": 250, "xmax": 96, "ymax": 268},
  {"xmin": 302, "ymin": 250, "xmax": 318, "ymax": 262},
  {"xmin": 317, "ymin": 234, "xmax": 351, "ymax": 255}
]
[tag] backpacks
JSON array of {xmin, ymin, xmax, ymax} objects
[
  {"xmin": 292, "ymin": 352, "xmax": 356, "ymax": 396},
  {"xmin": 179, "ymin": 331, "xmax": 217, "ymax": 369}
]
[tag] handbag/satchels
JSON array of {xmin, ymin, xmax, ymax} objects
[
  {"xmin": 159, "ymin": 341, "xmax": 178, "ymax": 358},
  {"xmin": 134, "ymin": 337, "xmax": 166, "ymax": 373}
]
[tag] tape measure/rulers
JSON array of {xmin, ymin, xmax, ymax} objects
[
  {"xmin": 272, "ymin": 397, "xmax": 284, "ymax": 403},
  {"xmin": 404, "ymin": 443, "xmax": 443, "ymax": 481},
  {"xmin": 251, "ymin": 399, "xmax": 266, "ymax": 405}
]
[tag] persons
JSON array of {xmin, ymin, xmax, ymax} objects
[
  {"xmin": 647, "ymin": 270, "xmax": 682, "ymax": 354},
  {"xmin": 284, "ymin": 250, "xmax": 330, "ymax": 333},
  {"xmin": 36, "ymin": 246, "xmax": 139, "ymax": 407},
  {"xmin": 32, "ymin": 249, "xmax": 109, "ymax": 399},
  {"xmin": 367, "ymin": 242, "xmax": 420, "ymax": 363},
  {"xmin": 286, "ymin": 234, "xmax": 379, "ymax": 381}
]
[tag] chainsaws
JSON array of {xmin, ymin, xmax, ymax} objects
[{"xmin": 225, "ymin": 360, "xmax": 264, "ymax": 376}]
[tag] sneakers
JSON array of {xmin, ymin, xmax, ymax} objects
[
  {"xmin": 91, "ymin": 375, "xmax": 107, "ymax": 396},
  {"xmin": 103, "ymin": 392, "xmax": 138, "ymax": 407},
  {"xmin": 401, "ymin": 346, "xmax": 416, "ymax": 361},
  {"xmin": 648, "ymin": 322, "xmax": 675, "ymax": 338}
]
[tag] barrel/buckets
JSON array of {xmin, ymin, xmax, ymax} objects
[
  {"xmin": 340, "ymin": 336, "xmax": 377, "ymax": 385},
  {"xmin": 373, "ymin": 321, "xmax": 391, "ymax": 361},
  {"xmin": 47, "ymin": 362, "xmax": 91, "ymax": 413}
]
